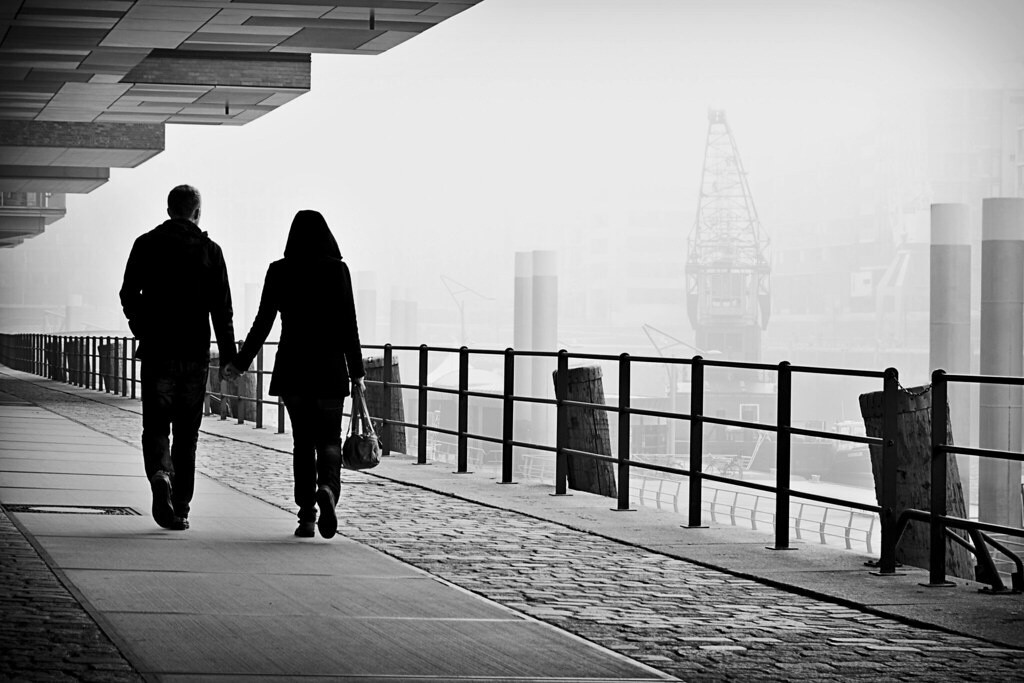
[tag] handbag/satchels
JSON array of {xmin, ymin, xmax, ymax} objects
[{"xmin": 342, "ymin": 386, "xmax": 383, "ymax": 470}]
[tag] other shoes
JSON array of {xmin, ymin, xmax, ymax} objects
[
  {"xmin": 175, "ymin": 517, "xmax": 189, "ymax": 530},
  {"xmin": 151, "ymin": 472, "xmax": 175, "ymax": 528},
  {"xmin": 295, "ymin": 523, "xmax": 314, "ymax": 537},
  {"xmin": 316, "ymin": 486, "xmax": 338, "ymax": 539}
]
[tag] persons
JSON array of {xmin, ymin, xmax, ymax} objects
[
  {"xmin": 119, "ymin": 184, "xmax": 237, "ymax": 531},
  {"xmin": 224, "ymin": 211, "xmax": 366, "ymax": 536}
]
